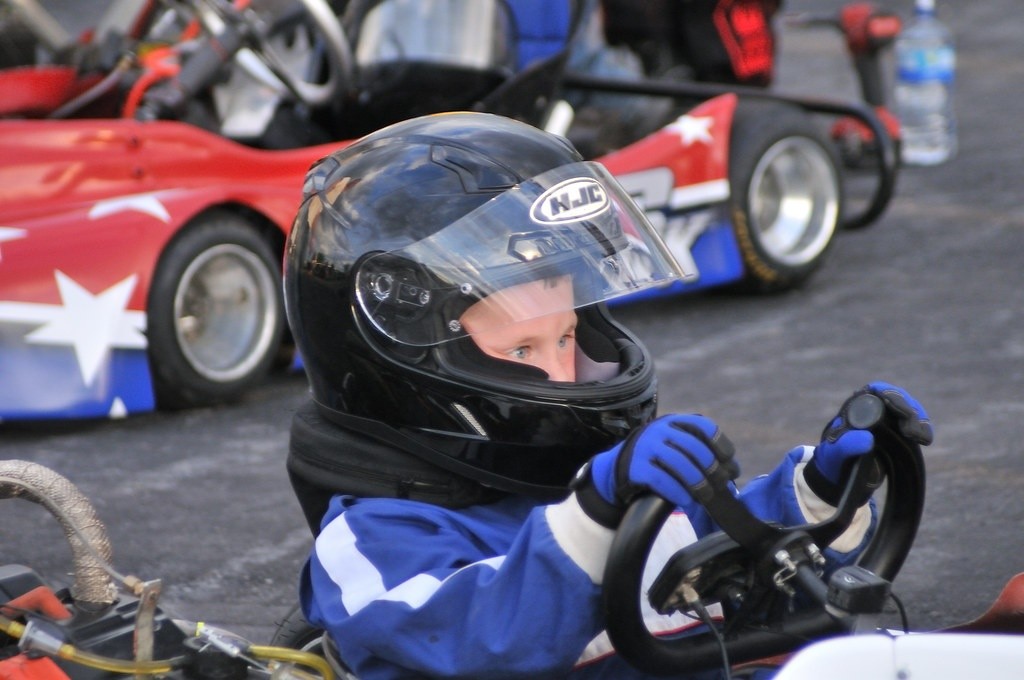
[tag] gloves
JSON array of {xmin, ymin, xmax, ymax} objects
[
  {"xmin": 803, "ymin": 383, "xmax": 934, "ymax": 510},
  {"xmin": 568, "ymin": 414, "xmax": 739, "ymax": 529}
]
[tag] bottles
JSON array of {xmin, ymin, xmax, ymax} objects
[{"xmin": 889, "ymin": 1, "xmax": 959, "ymax": 167}]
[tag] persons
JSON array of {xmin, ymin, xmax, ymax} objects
[
  {"xmin": 284, "ymin": 109, "xmax": 935, "ymax": 680},
  {"xmin": 597, "ymin": 0, "xmax": 784, "ymax": 91}
]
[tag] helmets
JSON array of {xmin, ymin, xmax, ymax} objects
[{"xmin": 283, "ymin": 111, "xmax": 657, "ymax": 498}]
